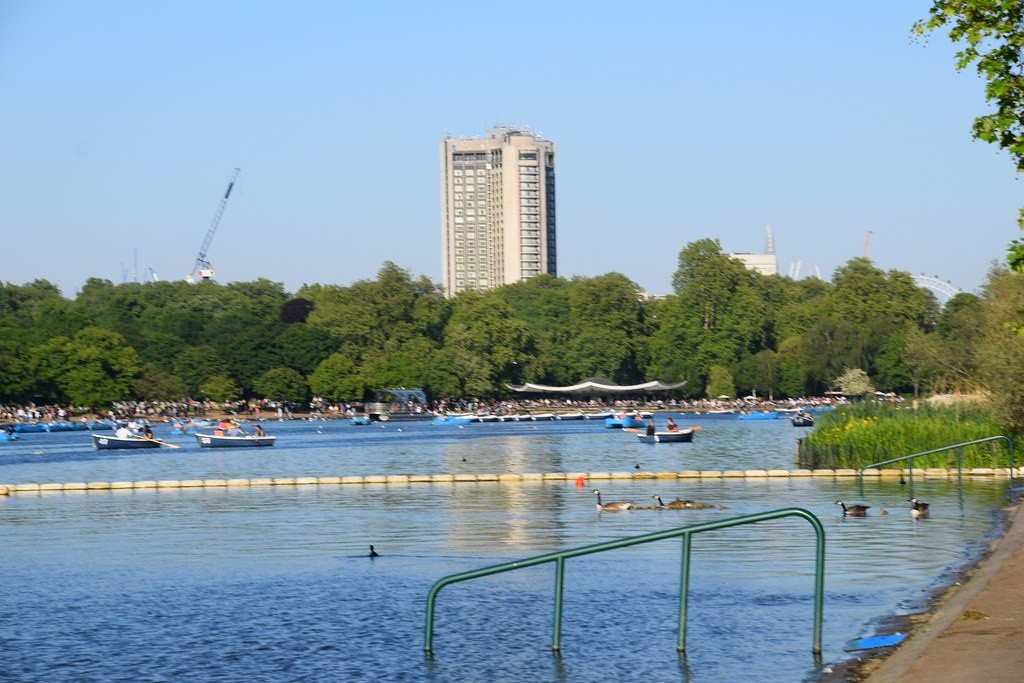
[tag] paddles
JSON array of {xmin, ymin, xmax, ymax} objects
[
  {"xmin": 132, "ymin": 434, "xmax": 180, "ymax": 448},
  {"xmin": 245, "ymin": 430, "xmax": 262, "ymax": 447},
  {"xmin": 663, "ymin": 426, "xmax": 702, "ymax": 431},
  {"xmin": 621, "ymin": 427, "xmax": 645, "ymax": 435}
]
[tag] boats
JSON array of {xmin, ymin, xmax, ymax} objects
[
  {"xmin": 653, "ymin": 427, "xmax": 696, "ymax": 443},
  {"xmin": 604, "ymin": 416, "xmax": 645, "ymax": 429},
  {"xmin": 557, "ymin": 413, "xmax": 584, "ymax": 420},
  {"xmin": 90, "ymin": 431, "xmax": 165, "ymax": 450},
  {"xmin": 455, "ymin": 415, "xmax": 480, "ymax": 422},
  {"xmin": 791, "ymin": 410, "xmax": 815, "ymax": 427},
  {"xmin": 479, "ymin": 415, "xmax": 499, "ymax": 422},
  {"xmin": 433, "ymin": 416, "xmax": 473, "ymax": 426},
  {"xmin": 512, "ymin": 414, "xmax": 531, "ymax": 421},
  {"xmin": 625, "ymin": 412, "xmax": 655, "ymax": 419},
  {"xmin": 584, "ymin": 412, "xmax": 613, "ymax": 420},
  {"xmin": 194, "ymin": 433, "xmax": 277, "ymax": 448},
  {"xmin": 497, "ymin": 414, "xmax": 514, "ymax": 421},
  {"xmin": 708, "ymin": 409, "xmax": 735, "ymax": 414},
  {"xmin": 774, "ymin": 406, "xmax": 804, "ymax": 415},
  {"xmin": 636, "ymin": 433, "xmax": 656, "ymax": 443},
  {"xmin": 530, "ymin": 412, "xmax": 556, "ymax": 421},
  {"xmin": 350, "ymin": 416, "xmax": 371, "ymax": 425},
  {"xmin": 0, "ymin": 421, "xmax": 154, "ymax": 444}
]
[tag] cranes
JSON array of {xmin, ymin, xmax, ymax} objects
[{"xmin": 192, "ymin": 168, "xmax": 242, "ymax": 279}]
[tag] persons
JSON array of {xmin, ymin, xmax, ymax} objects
[
  {"xmin": 646, "ymin": 420, "xmax": 655, "ymax": 436},
  {"xmin": 0, "ymin": 394, "xmax": 351, "ymax": 440},
  {"xmin": 797, "ymin": 411, "xmax": 813, "ymax": 420},
  {"xmin": 391, "ymin": 397, "xmax": 665, "ymax": 421},
  {"xmin": 665, "ymin": 396, "xmax": 850, "ymax": 409},
  {"xmin": 666, "ymin": 418, "xmax": 678, "ymax": 432}
]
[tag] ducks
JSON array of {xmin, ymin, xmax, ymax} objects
[
  {"xmin": 907, "ymin": 497, "xmax": 929, "ymax": 519},
  {"xmin": 880, "ymin": 507, "xmax": 888, "ymax": 515},
  {"xmin": 591, "ymin": 489, "xmax": 637, "ymax": 512},
  {"xmin": 634, "ymin": 464, "xmax": 640, "ymax": 469},
  {"xmin": 898, "ymin": 474, "xmax": 907, "ymax": 484},
  {"xmin": 369, "ymin": 545, "xmax": 379, "ymax": 556},
  {"xmin": 651, "ymin": 494, "xmax": 728, "ymax": 510},
  {"xmin": 0, "ymin": 486, "xmax": 15, "ymax": 496},
  {"xmin": 32, "ymin": 449, "xmax": 48, "ymax": 455},
  {"xmin": 833, "ymin": 500, "xmax": 871, "ymax": 516}
]
